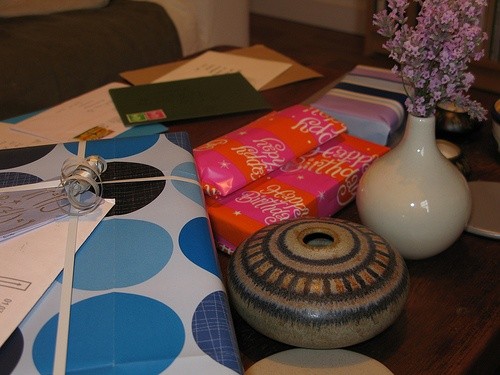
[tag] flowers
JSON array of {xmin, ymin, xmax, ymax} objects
[{"xmin": 373, "ymin": 0, "xmax": 492, "ymax": 123}]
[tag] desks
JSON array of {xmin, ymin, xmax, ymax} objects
[{"xmin": 153, "ymin": 44, "xmax": 499, "ymax": 375}]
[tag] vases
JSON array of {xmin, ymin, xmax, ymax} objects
[{"xmin": 355, "ymin": 113, "xmax": 473, "ymax": 261}]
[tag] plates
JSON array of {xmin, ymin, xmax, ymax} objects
[{"xmin": 466, "ymin": 180, "xmax": 500, "ymax": 240}]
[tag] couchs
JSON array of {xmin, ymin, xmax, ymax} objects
[{"xmin": 0, "ymin": 0, "xmax": 183, "ymax": 120}]
